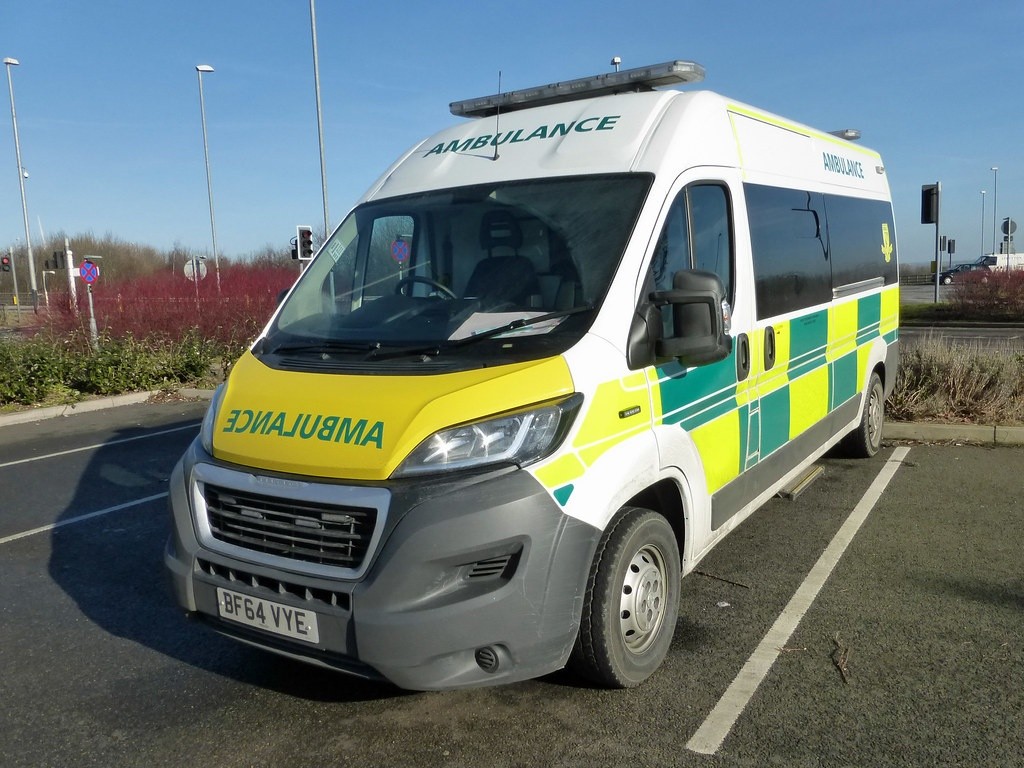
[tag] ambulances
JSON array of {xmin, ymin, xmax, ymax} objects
[{"xmin": 159, "ymin": 56, "xmax": 903, "ymax": 693}]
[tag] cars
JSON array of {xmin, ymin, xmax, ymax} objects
[{"xmin": 932, "ymin": 262, "xmax": 975, "ymax": 284}]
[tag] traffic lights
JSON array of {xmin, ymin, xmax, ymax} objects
[
  {"xmin": 2, "ymin": 253, "xmax": 11, "ymax": 273},
  {"xmin": 37, "ymin": 248, "xmax": 67, "ymax": 273},
  {"xmin": 288, "ymin": 225, "xmax": 315, "ymax": 260}
]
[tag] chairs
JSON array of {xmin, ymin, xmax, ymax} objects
[
  {"xmin": 461, "ymin": 208, "xmax": 537, "ymax": 312},
  {"xmin": 534, "ymin": 199, "xmax": 659, "ymax": 315}
]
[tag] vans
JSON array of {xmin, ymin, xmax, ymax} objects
[{"xmin": 974, "ymin": 254, "xmax": 1023, "ymax": 276}]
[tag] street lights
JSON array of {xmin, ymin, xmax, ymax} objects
[
  {"xmin": 990, "ymin": 166, "xmax": 998, "ymax": 255},
  {"xmin": 188, "ymin": 59, "xmax": 215, "ymax": 284},
  {"xmin": 186, "ymin": 61, "xmax": 216, "ymax": 285},
  {"xmin": 979, "ymin": 189, "xmax": 987, "ymax": 257},
  {"xmin": 3, "ymin": 54, "xmax": 43, "ymax": 318}
]
